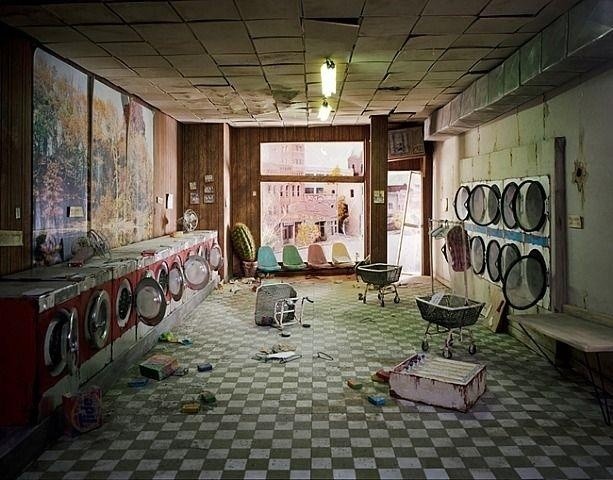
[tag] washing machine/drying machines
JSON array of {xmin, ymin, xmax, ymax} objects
[{"xmin": 0, "ymin": 231, "xmax": 223, "ymax": 430}]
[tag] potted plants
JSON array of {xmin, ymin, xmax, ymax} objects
[{"xmin": 231, "ymin": 222, "xmax": 258, "ymax": 278}]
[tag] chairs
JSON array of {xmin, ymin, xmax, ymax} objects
[{"xmin": 257, "ymin": 243, "xmax": 357, "ymax": 276}]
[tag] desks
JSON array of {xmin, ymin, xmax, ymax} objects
[{"xmin": 507, "ymin": 314, "xmax": 613, "ymax": 427}]
